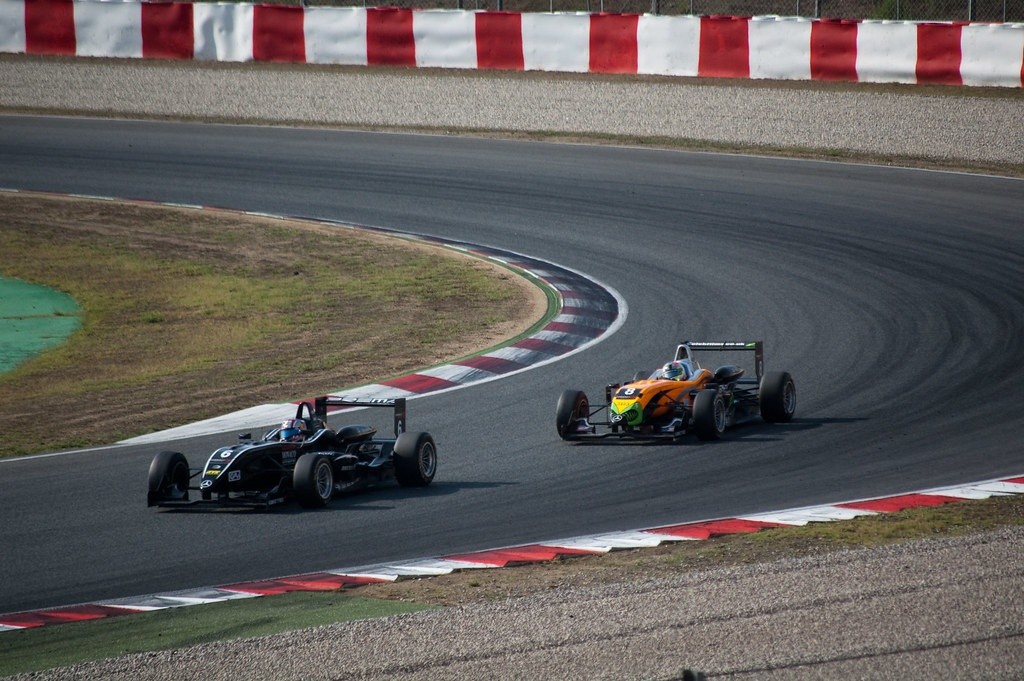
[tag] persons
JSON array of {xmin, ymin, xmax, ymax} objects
[
  {"xmin": 280, "ymin": 419, "xmax": 306, "ymax": 442},
  {"xmin": 662, "ymin": 363, "xmax": 687, "ymax": 381}
]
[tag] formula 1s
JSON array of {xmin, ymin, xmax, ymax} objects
[
  {"xmin": 146, "ymin": 396, "xmax": 437, "ymax": 513},
  {"xmin": 555, "ymin": 339, "xmax": 797, "ymax": 443}
]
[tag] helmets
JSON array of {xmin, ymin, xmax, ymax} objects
[
  {"xmin": 662, "ymin": 361, "xmax": 686, "ymax": 381},
  {"xmin": 279, "ymin": 419, "xmax": 307, "ymax": 441}
]
